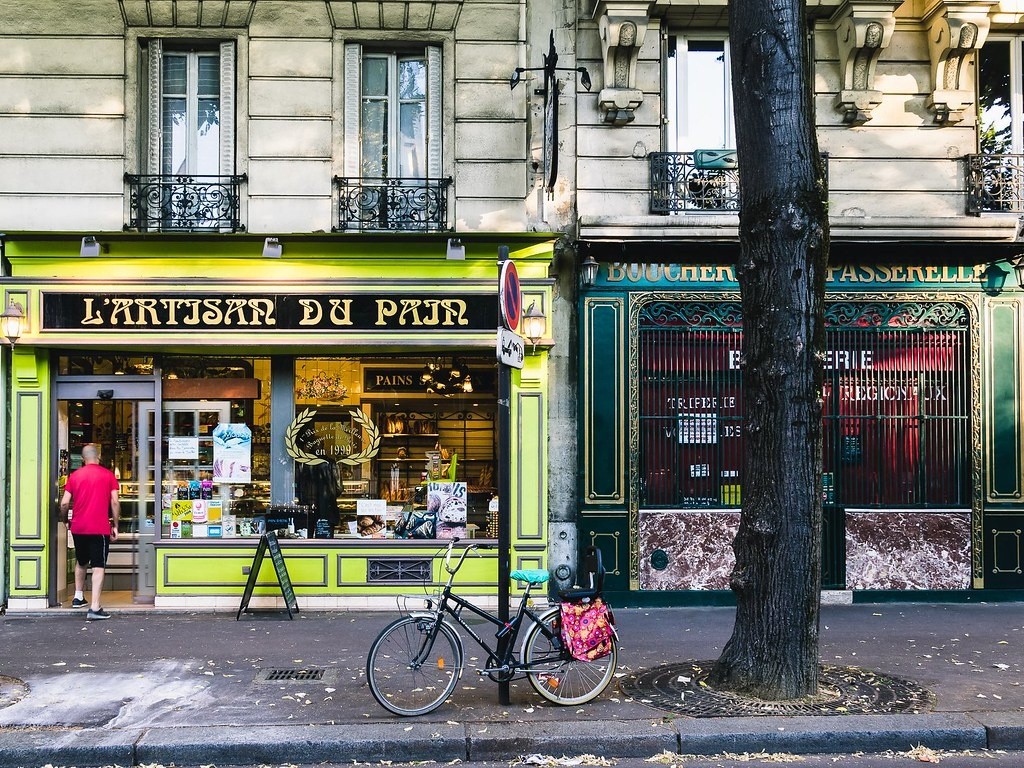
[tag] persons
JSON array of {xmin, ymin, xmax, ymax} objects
[
  {"xmin": 298, "ymin": 436, "xmax": 344, "ymax": 526},
  {"xmin": 60, "ymin": 444, "xmax": 121, "ymax": 618}
]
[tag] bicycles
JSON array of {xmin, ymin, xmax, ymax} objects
[{"xmin": 365, "ymin": 537, "xmax": 619, "ymax": 718}]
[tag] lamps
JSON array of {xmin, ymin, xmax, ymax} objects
[
  {"xmin": 0, "ymin": 298, "xmax": 24, "ymax": 353},
  {"xmin": 987, "ymin": 253, "xmax": 1024, "ymax": 290},
  {"xmin": 420, "ymin": 353, "xmax": 475, "ymax": 397},
  {"xmin": 555, "ymin": 67, "xmax": 591, "ymax": 92},
  {"xmin": 262, "ymin": 238, "xmax": 283, "ymax": 258},
  {"xmin": 510, "ymin": 67, "xmax": 548, "ymax": 90},
  {"xmin": 581, "ymin": 256, "xmax": 599, "ymax": 289},
  {"xmin": 80, "ymin": 237, "xmax": 107, "ymax": 257},
  {"xmin": 447, "ymin": 236, "xmax": 466, "ymax": 260},
  {"xmin": 114, "ymin": 357, "xmax": 178, "ymax": 379}
]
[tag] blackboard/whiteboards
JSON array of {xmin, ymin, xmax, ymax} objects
[{"xmin": 264, "ymin": 530, "xmax": 300, "ymax": 612}]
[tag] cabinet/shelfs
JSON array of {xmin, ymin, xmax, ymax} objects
[{"xmin": 377, "ymin": 407, "xmax": 497, "ymax": 495}]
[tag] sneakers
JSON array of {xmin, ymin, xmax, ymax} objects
[
  {"xmin": 72, "ymin": 596, "xmax": 89, "ymax": 607},
  {"xmin": 87, "ymin": 607, "xmax": 111, "ymax": 619}
]
